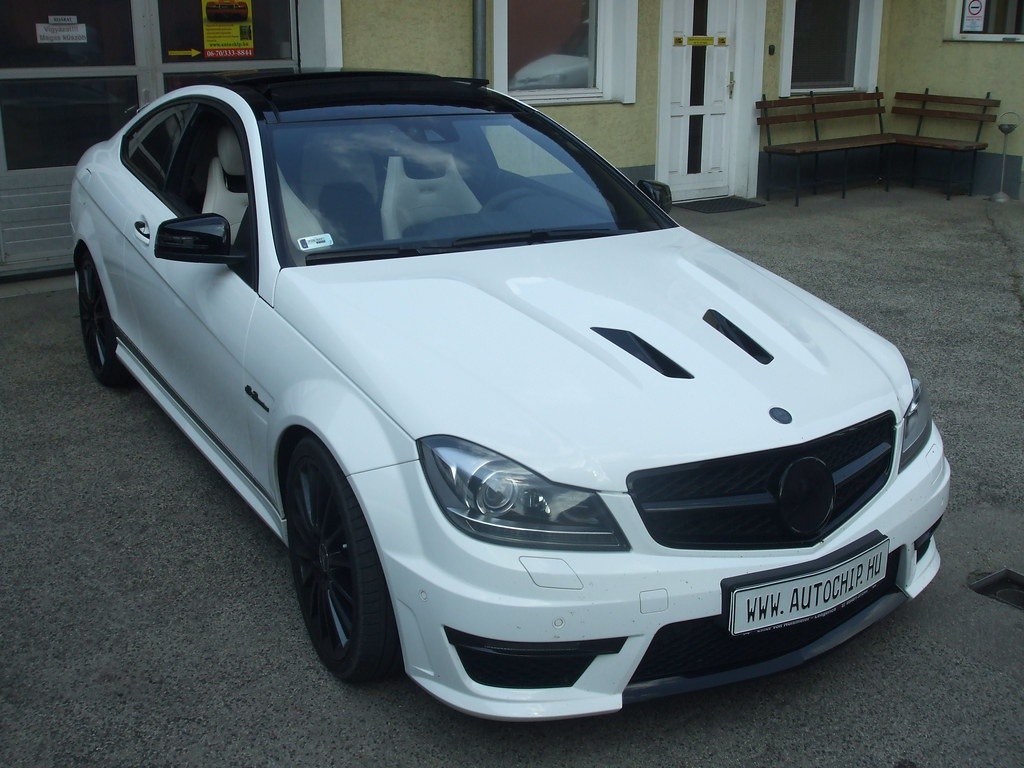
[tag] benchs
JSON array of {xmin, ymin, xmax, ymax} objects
[
  {"xmin": 889, "ymin": 88, "xmax": 1001, "ymax": 200},
  {"xmin": 756, "ymin": 86, "xmax": 896, "ymax": 207}
]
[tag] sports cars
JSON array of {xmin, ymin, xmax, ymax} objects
[{"xmin": 67, "ymin": 65, "xmax": 949, "ymax": 720}]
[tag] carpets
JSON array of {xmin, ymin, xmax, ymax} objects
[{"xmin": 672, "ymin": 196, "xmax": 766, "ymax": 213}]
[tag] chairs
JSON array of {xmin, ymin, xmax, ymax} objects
[
  {"xmin": 202, "ymin": 125, "xmax": 331, "ymax": 267},
  {"xmin": 380, "ymin": 124, "xmax": 482, "ymax": 241}
]
